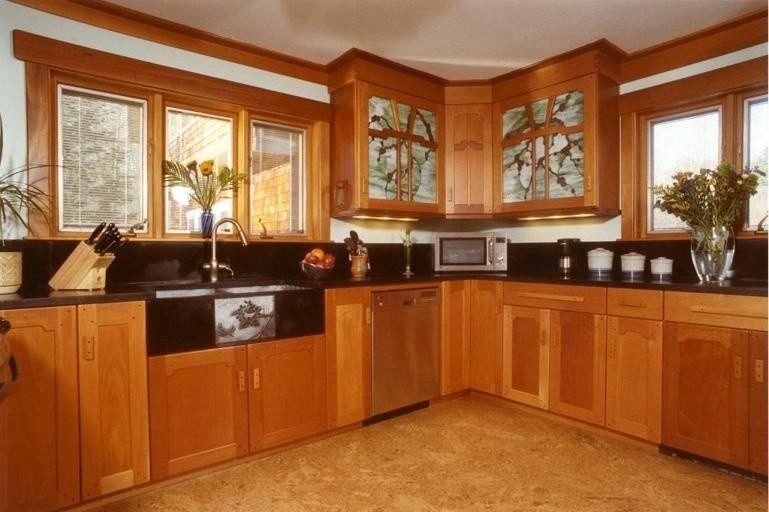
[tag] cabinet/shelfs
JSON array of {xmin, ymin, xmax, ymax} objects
[
  {"xmin": 0, "ymin": 304, "xmax": 80, "ymax": 512},
  {"xmin": 470, "ymin": 278, "xmax": 502, "ymax": 401},
  {"xmin": 501, "ymin": 279, "xmax": 606, "ymax": 433},
  {"xmin": 147, "ymin": 332, "xmax": 328, "ymax": 489},
  {"xmin": 488, "ymin": 49, "xmax": 622, "ymax": 220},
  {"xmin": 323, "ymin": 285, "xmax": 374, "ymax": 438},
  {"xmin": 603, "ymin": 284, "xmax": 664, "ymax": 456},
  {"xmin": 328, "ymin": 56, "xmax": 448, "ymax": 222},
  {"xmin": 440, "ymin": 280, "xmax": 471, "ymax": 400},
  {"xmin": 78, "ymin": 296, "xmax": 153, "ymax": 510},
  {"xmin": 442, "ymin": 83, "xmax": 495, "ymax": 221},
  {"xmin": 661, "ymin": 288, "xmax": 768, "ymax": 486}
]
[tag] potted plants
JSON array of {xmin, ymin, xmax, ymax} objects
[{"xmin": 0, "ymin": 112, "xmax": 76, "ymax": 294}]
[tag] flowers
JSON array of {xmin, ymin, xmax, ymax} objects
[
  {"xmin": 161, "ymin": 156, "xmax": 251, "ymax": 209},
  {"xmin": 344, "ymin": 230, "xmax": 369, "ymax": 261},
  {"xmin": 649, "ymin": 160, "xmax": 767, "ymax": 267}
]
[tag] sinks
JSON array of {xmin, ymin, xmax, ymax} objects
[
  {"xmin": 218, "ymin": 284, "xmax": 312, "ymax": 295},
  {"xmin": 154, "ymin": 286, "xmax": 219, "ymax": 299}
]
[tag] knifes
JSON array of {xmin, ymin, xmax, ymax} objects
[{"xmin": 86, "ymin": 221, "xmax": 128, "ymax": 256}]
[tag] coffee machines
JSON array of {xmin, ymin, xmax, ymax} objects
[{"xmin": 556, "ymin": 237, "xmax": 588, "ymax": 281}]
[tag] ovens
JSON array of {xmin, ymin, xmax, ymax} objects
[{"xmin": 369, "ymin": 287, "xmax": 441, "ymax": 417}]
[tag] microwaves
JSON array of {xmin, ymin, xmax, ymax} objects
[{"xmin": 432, "ymin": 234, "xmax": 507, "ymax": 272}]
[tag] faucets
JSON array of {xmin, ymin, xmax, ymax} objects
[{"xmin": 209, "ymin": 218, "xmax": 249, "ymax": 282}]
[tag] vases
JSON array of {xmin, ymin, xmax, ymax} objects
[
  {"xmin": 201, "ymin": 208, "xmax": 214, "ymax": 240},
  {"xmin": 351, "ymin": 254, "xmax": 369, "ymax": 278},
  {"xmin": 690, "ymin": 221, "xmax": 737, "ymax": 284}
]
